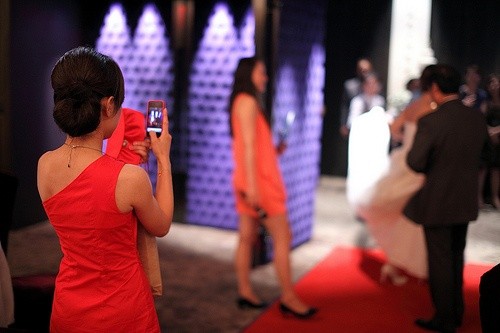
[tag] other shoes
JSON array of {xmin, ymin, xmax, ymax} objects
[{"xmin": 416, "ymin": 316, "xmax": 459, "ymax": 333}]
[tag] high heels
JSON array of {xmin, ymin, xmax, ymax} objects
[
  {"xmin": 236, "ymin": 295, "xmax": 270, "ymax": 311},
  {"xmin": 379, "ymin": 266, "xmax": 407, "ymax": 287},
  {"xmin": 278, "ymin": 302, "xmax": 318, "ymax": 320}
]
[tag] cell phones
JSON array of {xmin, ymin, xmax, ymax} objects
[{"xmin": 145, "ymin": 100, "xmax": 167, "ymax": 142}]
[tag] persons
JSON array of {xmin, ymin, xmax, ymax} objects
[
  {"xmin": 341, "ymin": 59, "xmax": 437, "ymax": 287},
  {"xmin": 456, "ymin": 62, "xmax": 500, "ymax": 214},
  {"xmin": 401, "ymin": 61, "xmax": 494, "ymax": 333},
  {"xmin": 225, "ymin": 55, "xmax": 319, "ymax": 320},
  {"xmin": 38, "ymin": 47, "xmax": 174, "ymax": 333}
]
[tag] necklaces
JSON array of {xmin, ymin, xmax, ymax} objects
[{"xmin": 64, "ymin": 142, "xmax": 106, "ymax": 168}]
